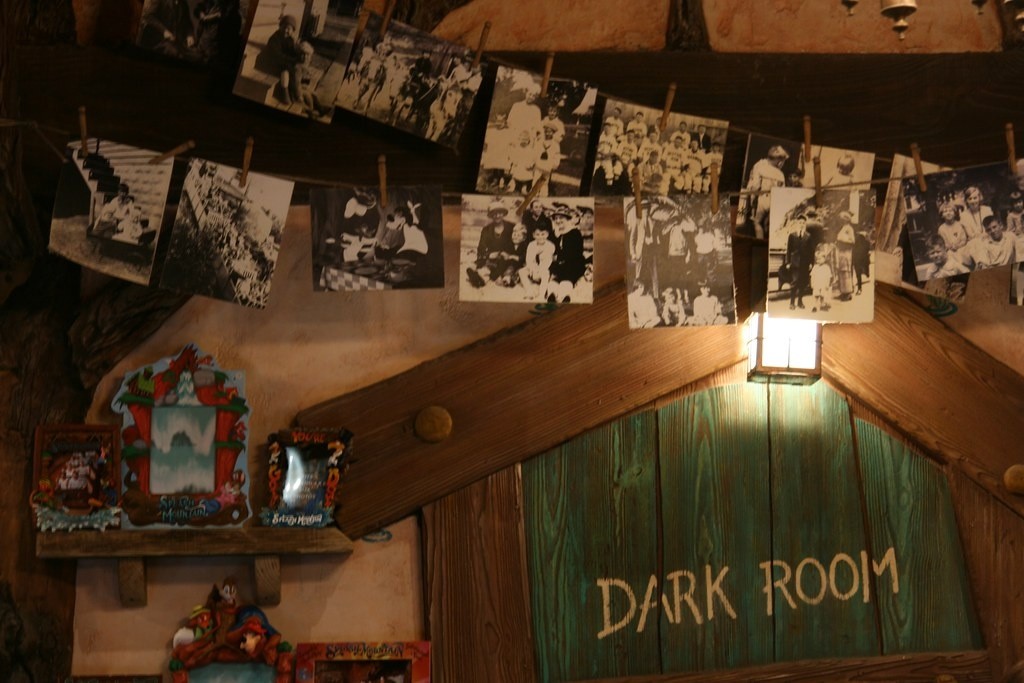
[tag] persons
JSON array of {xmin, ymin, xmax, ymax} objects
[
  {"xmin": 53, "ymin": 449, "xmax": 111, "ymax": 515},
  {"xmin": 90, "ymin": 8, "xmax": 1023, "ymax": 329}
]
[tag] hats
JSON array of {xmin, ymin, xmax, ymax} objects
[
  {"xmin": 806, "ymin": 210, "xmax": 817, "ymax": 215},
  {"xmin": 575, "ymin": 205, "xmax": 594, "ymax": 219},
  {"xmin": 487, "ymin": 202, "xmax": 508, "ymax": 219},
  {"xmin": 546, "ymin": 206, "xmax": 572, "ymax": 220},
  {"xmin": 280, "ymin": 16, "xmax": 296, "ymax": 31}
]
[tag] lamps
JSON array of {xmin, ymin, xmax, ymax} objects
[{"xmin": 744, "ymin": 312, "xmax": 823, "ymax": 386}]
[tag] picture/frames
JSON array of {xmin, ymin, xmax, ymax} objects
[
  {"xmin": 109, "ymin": 342, "xmax": 254, "ymax": 528},
  {"xmin": 259, "ymin": 426, "xmax": 351, "ymax": 528},
  {"xmin": 169, "ymin": 582, "xmax": 292, "ymax": 682},
  {"xmin": 296, "ymin": 640, "xmax": 431, "ymax": 683},
  {"xmin": 29, "ymin": 423, "xmax": 122, "ymax": 531}
]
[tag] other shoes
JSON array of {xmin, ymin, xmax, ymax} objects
[
  {"xmin": 798, "ymin": 302, "xmax": 804, "ymax": 308},
  {"xmin": 562, "ymin": 296, "xmax": 572, "ymax": 303},
  {"xmin": 547, "ymin": 292, "xmax": 557, "ymax": 303},
  {"xmin": 835, "ymin": 293, "xmax": 851, "ymax": 301},
  {"xmin": 466, "ymin": 268, "xmax": 485, "ymax": 287},
  {"xmin": 790, "ymin": 303, "xmax": 795, "ymax": 310}
]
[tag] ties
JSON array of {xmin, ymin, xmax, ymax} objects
[{"xmin": 799, "ymin": 230, "xmax": 802, "ymax": 238}]
